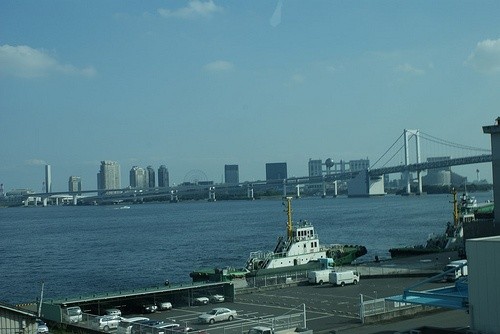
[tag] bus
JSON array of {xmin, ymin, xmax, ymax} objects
[{"xmin": 118, "ymin": 317, "xmax": 149, "ymax": 334}]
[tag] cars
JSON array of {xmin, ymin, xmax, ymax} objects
[
  {"xmin": 199, "ymin": 307, "xmax": 238, "ymax": 325},
  {"xmin": 35, "ymin": 319, "xmax": 49, "ymax": 334},
  {"xmin": 248, "ymin": 326, "xmax": 274, "ymax": 334},
  {"xmin": 131, "ymin": 320, "xmax": 192, "ymax": 334},
  {"xmin": 206, "ymin": 290, "xmax": 225, "ymax": 303},
  {"xmin": 182, "ymin": 292, "xmax": 209, "ymax": 306},
  {"xmin": 97, "ymin": 316, "xmax": 125, "ymax": 332},
  {"xmin": 139, "ymin": 301, "xmax": 158, "ymax": 313},
  {"xmin": 158, "ymin": 301, "xmax": 172, "ymax": 310},
  {"xmin": 105, "ymin": 307, "xmax": 122, "ymax": 316}
]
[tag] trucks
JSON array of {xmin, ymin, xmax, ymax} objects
[
  {"xmin": 328, "ymin": 271, "xmax": 361, "ymax": 286},
  {"xmin": 446, "ymin": 259, "xmax": 468, "ymax": 280},
  {"xmin": 308, "ymin": 270, "xmax": 336, "ymax": 285},
  {"xmin": 62, "ymin": 306, "xmax": 83, "ymax": 323}
]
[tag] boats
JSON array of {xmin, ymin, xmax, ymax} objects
[
  {"xmin": 388, "ymin": 188, "xmax": 494, "ymax": 258},
  {"xmin": 188, "ymin": 196, "xmax": 367, "ymax": 282}
]
